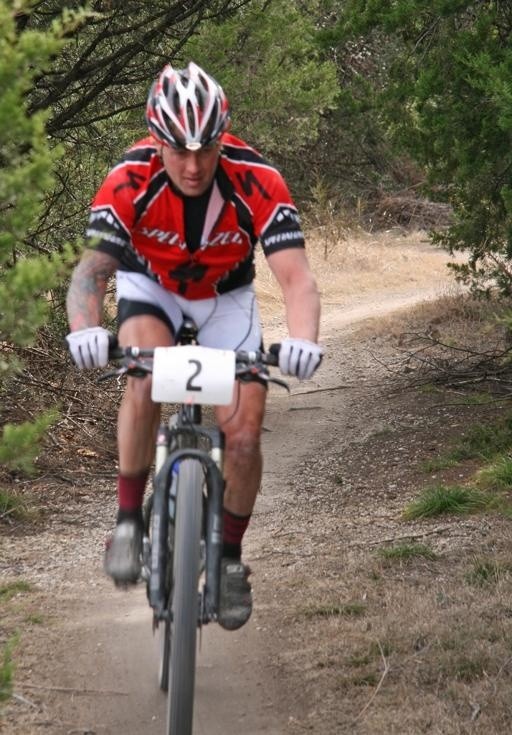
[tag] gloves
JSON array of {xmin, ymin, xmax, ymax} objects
[
  {"xmin": 269, "ymin": 338, "xmax": 325, "ymax": 381},
  {"xmin": 65, "ymin": 326, "xmax": 118, "ymax": 370}
]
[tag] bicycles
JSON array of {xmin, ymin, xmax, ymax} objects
[{"xmin": 69, "ymin": 314, "xmax": 309, "ymax": 735}]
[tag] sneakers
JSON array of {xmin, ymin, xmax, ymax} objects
[
  {"xmin": 218, "ymin": 557, "xmax": 252, "ymax": 630},
  {"xmin": 105, "ymin": 519, "xmax": 146, "ymax": 583}
]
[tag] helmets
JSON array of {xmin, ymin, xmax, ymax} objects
[{"xmin": 145, "ymin": 62, "xmax": 233, "ymax": 152}]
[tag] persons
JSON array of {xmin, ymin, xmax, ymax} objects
[{"xmin": 65, "ymin": 55, "xmax": 325, "ymax": 636}]
